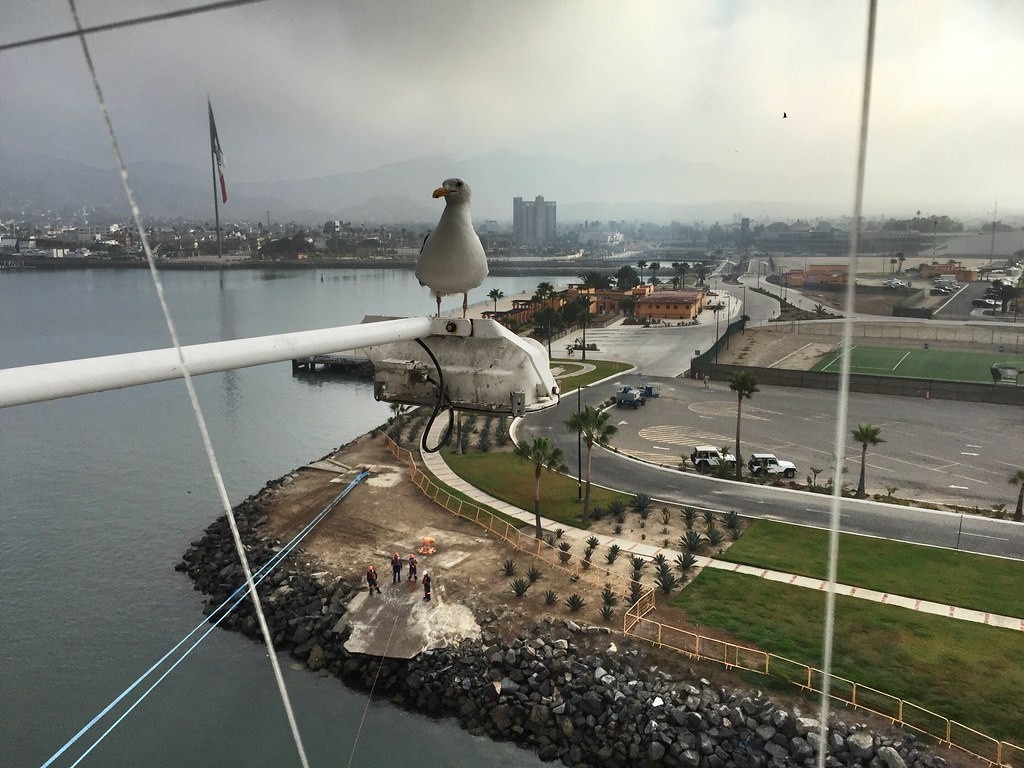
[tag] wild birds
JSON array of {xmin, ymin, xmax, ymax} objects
[
  {"xmin": 783, "ymin": 112, "xmax": 787, "ymax": 118},
  {"xmin": 413, "ymin": 176, "xmax": 489, "ymax": 318}
]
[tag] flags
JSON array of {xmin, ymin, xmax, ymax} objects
[{"xmin": 210, "ymin": 105, "xmax": 227, "ymax": 204}]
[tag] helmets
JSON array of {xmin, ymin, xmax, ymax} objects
[
  {"xmin": 368, "ymin": 566, "xmax": 373, "ymax": 570},
  {"xmin": 410, "ymin": 554, "xmax": 414, "ymax": 558},
  {"xmin": 395, "ymin": 552, "xmax": 399, "ymax": 556},
  {"xmin": 423, "ymin": 571, "xmax": 427, "ymax": 575}
]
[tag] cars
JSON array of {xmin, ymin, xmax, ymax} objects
[{"xmin": 881, "ymin": 274, "xmax": 1004, "ymax": 309}]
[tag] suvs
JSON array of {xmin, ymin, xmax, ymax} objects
[
  {"xmin": 690, "ymin": 446, "xmax": 737, "ymax": 472},
  {"xmin": 748, "ymin": 454, "xmax": 797, "ymax": 479}
]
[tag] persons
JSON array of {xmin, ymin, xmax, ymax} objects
[
  {"xmin": 408, "ymin": 554, "xmax": 418, "ymax": 582},
  {"xmin": 367, "ymin": 566, "xmax": 382, "ymax": 595},
  {"xmin": 391, "ymin": 552, "xmax": 402, "ymax": 584},
  {"xmin": 422, "ymin": 571, "xmax": 431, "ymax": 602}
]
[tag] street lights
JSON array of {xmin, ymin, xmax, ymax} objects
[
  {"xmin": 724, "ymin": 295, "xmax": 730, "ymax": 350},
  {"xmin": 738, "ymin": 286, "xmax": 745, "ymax": 316},
  {"xmin": 578, "ymin": 385, "xmax": 592, "ymax": 500}
]
